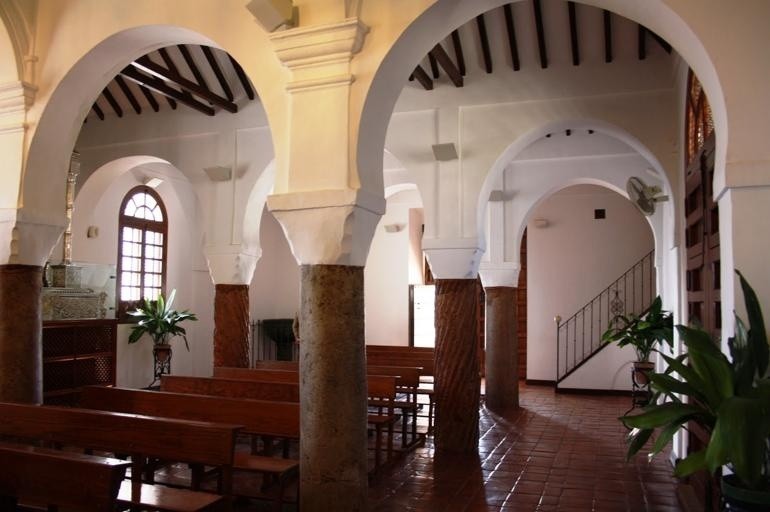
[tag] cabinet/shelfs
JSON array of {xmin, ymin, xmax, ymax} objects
[{"xmin": 43, "ymin": 318, "xmax": 119, "ymax": 407}]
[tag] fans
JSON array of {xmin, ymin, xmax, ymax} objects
[{"xmin": 625, "ymin": 176, "xmax": 668, "ymax": 217}]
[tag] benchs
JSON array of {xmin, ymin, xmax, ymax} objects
[{"xmin": 1, "ymin": 343, "xmax": 485, "ymax": 512}]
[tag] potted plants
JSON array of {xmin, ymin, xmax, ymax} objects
[
  {"xmin": 126, "ymin": 288, "xmax": 199, "ymax": 362},
  {"xmin": 617, "ymin": 268, "xmax": 770, "ymax": 512},
  {"xmin": 600, "ymin": 295, "xmax": 673, "ymax": 385}
]
